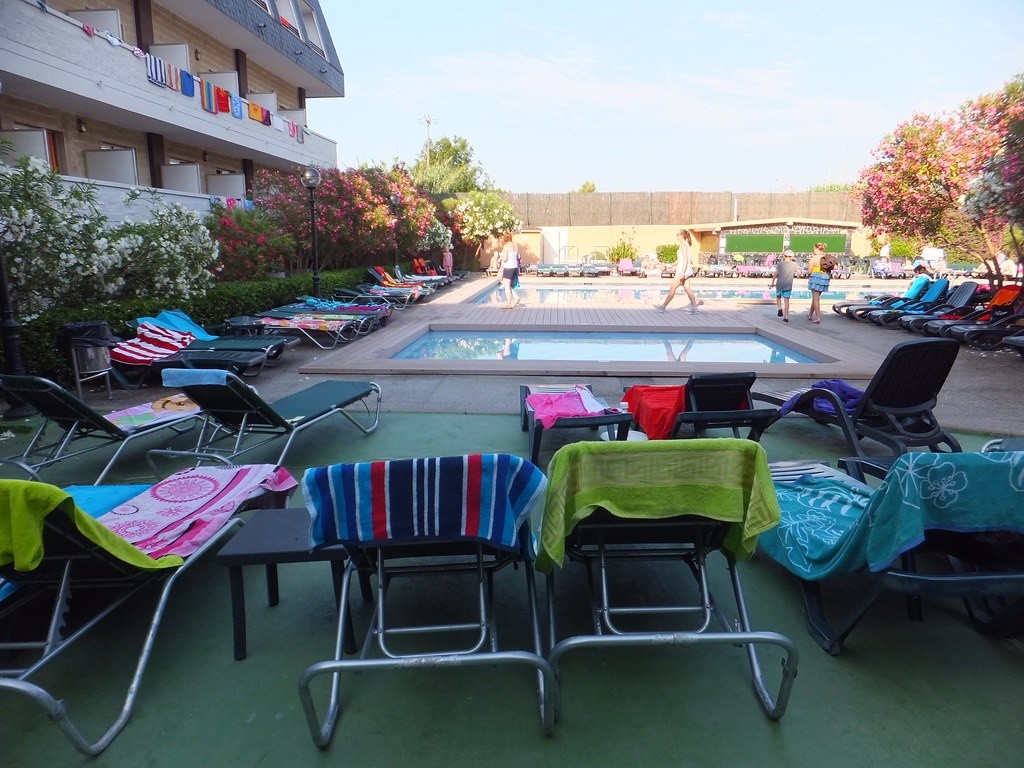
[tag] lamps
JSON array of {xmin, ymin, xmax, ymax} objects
[
  {"xmin": 195, "ymin": 49, "xmax": 201, "ymax": 60},
  {"xmin": 202, "ymin": 151, "xmax": 210, "ymax": 161},
  {"xmin": 77, "ymin": 118, "xmax": 87, "ymax": 132}
]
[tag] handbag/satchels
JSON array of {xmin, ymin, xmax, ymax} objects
[{"xmin": 821, "ymin": 252, "xmax": 839, "ymax": 274}]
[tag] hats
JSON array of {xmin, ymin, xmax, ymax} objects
[{"xmin": 783, "ymin": 250, "xmax": 795, "ymax": 257}]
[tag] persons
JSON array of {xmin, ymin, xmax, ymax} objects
[
  {"xmin": 638, "ymin": 255, "xmax": 649, "ymax": 278},
  {"xmin": 653, "ymin": 229, "xmax": 700, "ymax": 314},
  {"xmin": 806, "ymin": 242, "xmax": 830, "ymax": 324},
  {"xmin": 442, "ymin": 246, "xmax": 453, "ymax": 276},
  {"xmin": 499, "ymin": 231, "xmax": 520, "ymax": 309},
  {"xmin": 772, "ymin": 249, "xmax": 801, "ymax": 322},
  {"xmin": 496, "ymin": 338, "xmax": 520, "ymax": 360}
]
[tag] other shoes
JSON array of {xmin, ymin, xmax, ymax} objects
[
  {"xmin": 813, "ymin": 320, "xmax": 820, "ymax": 323},
  {"xmin": 778, "ymin": 308, "xmax": 783, "ymax": 316},
  {"xmin": 782, "ymin": 318, "xmax": 788, "ymax": 322},
  {"xmin": 688, "ymin": 309, "xmax": 700, "ymax": 315},
  {"xmin": 807, "ymin": 313, "xmax": 812, "ymax": 320},
  {"xmin": 653, "ymin": 304, "xmax": 665, "ymax": 313}
]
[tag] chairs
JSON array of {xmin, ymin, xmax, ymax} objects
[{"xmin": 0, "ymin": 252, "xmax": 1024, "ymax": 768}]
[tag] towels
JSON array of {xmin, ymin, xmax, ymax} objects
[
  {"xmin": 939, "ymin": 285, "xmax": 1024, "ymax": 321},
  {"xmin": 889, "ymin": 276, "xmax": 930, "ymax": 309},
  {"xmin": 161, "ymin": 368, "xmax": 230, "ymax": 388},
  {"xmin": 200, "ymin": 77, "xmax": 230, "ymax": 115},
  {"xmin": 109, "ymin": 320, "xmax": 196, "ymax": 366},
  {"xmin": 868, "ymin": 299, "xmax": 883, "ymax": 306},
  {"xmin": 230, "ymin": 92, "xmax": 243, "ymax": 120},
  {"xmin": 248, "ymin": 99, "xmax": 271, "ymax": 127},
  {"xmin": 535, "ymin": 437, "xmax": 781, "ymax": 576},
  {"xmin": 524, "ymin": 384, "xmax": 623, "ymax": 431},
  {"xmin": 902, "ymin": 278, "xmax": 949, "ymax": 315},
  {"xmin": 301, "ymin": 453, "xmax": 549, "ymax": 561},
  {"xmin": 95, "ymin": 464, "xmax": 299, "ymax": 560},
  {"xmin": 781, "ymin": 378, "xmax": 865, "ymax": 418},
  {"xmin": 145, "ymin": 52, "xmax": 194, "ymax": 98},
  {"xmin": 0, "ymin": 478, "xmax": 185, "ymax": 570},
  {"xmin": 620, "ymin": 383, "xmax": 686, "ymax": 440},
  {"xmin": 137, "ymin": 308, "xmax": 220, "ymax": 342},
  {"xmin": 751, "ymin": 451, "xmax": 1024, "ymax": 581},
  {"xmin": 101, "ymin": 392, "xmax": 201, "ymax": 432}
]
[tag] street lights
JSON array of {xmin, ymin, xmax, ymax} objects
[
  {"xmin": 299, "ymin": 167, "xmax": 324, "ymax": 299},
  {"xmin": 389, "ymin": 194, "xmax": 400, "ymax": 278}
]
